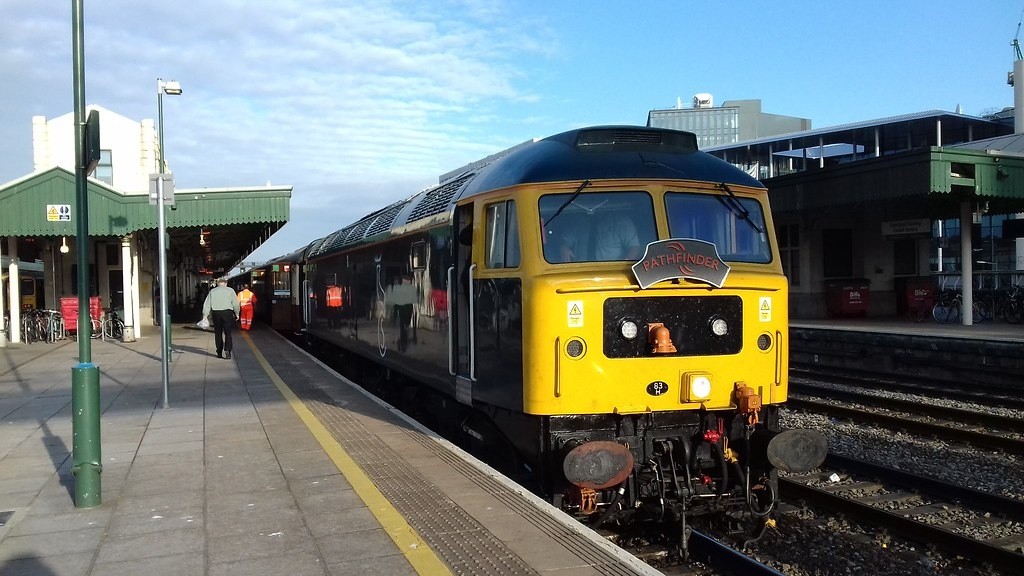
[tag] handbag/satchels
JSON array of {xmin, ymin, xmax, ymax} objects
[{"xmin": 197, "ymin": 317, "xmax": 209, "ymax": 330}]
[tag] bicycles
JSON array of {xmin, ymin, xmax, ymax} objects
[
  {"xmin": 3, "ymin": 304, "xmax": 62, "ymax": 345},
  {"xmin": 90, "ymin": 305, "xmax": 124, "ymax": 339},
  {"xmin": 910, "ymin": 273, "xmax": 1024, "ymax": 325}
]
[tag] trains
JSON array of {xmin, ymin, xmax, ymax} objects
[{"xmin": 226, "ymin": 123, "xmax": 827, "ymax": 563}]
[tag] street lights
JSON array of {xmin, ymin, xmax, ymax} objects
[{"xmin": 156, "ymin": 76, "xmax": 184, "ymax": 413}]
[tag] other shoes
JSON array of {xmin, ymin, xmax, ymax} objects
[
  {"xmin": 225, "ymin": 351, "xmax": 231, "ymax": 359},
  {"xmin": 217, "ymin": 352, "xmax": 222, "ymax": 358}
]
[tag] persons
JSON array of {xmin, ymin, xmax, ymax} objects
[
  {"xmin": 556, "ymin": 211, "xmax": 640, "ymax": 263},
  {"xmin": 326, "ymin": 286, "xmax": 343, "ymax": 331},
  {"xmin": 202, "ymin": 278, "xmax": 240, "ymax": 359},
  {"xmin": 238, "ymin": 284, "xmax": 257, "ymax": 332},
  {"xmin": 196, "ymin": 284, "xmax": 207, "ymax": 295},
  {"xmin": 395, "ymin": 275, "xmax": 416, "ymax": 352}
]
